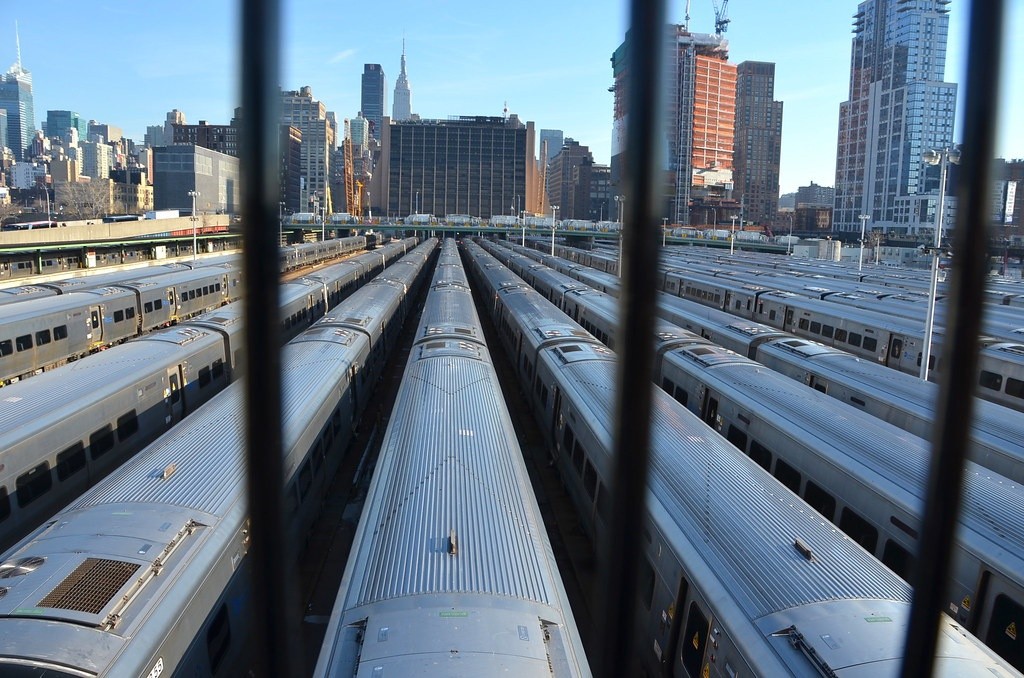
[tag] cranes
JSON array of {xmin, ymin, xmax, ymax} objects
[{"xmin": 714, "ymin": 1, "xmax": 732, "ymax": 34}]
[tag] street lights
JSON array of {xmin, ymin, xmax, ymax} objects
[
  {"xmin": 314, "ymin": 190, "xmax": 317, "ymax": 222},
  {"xmin": 874, "ymin": 233, "xmax": 881, "ymax": 266},
  {"xmin": 786, "ymin": 215, "xmax": 793, "ymax": 254},
  {"xmin": 415, "ymin": 192, "xmax": 419, "ymax": 214},
  {"xmin": 187, "ymin": 189, "xmax": 201, "ymax": 261},
  {"xmin": 366, "ymin": 191, "xmax": 372, "ymax": 221},
  {"xmin": 31, "ymin": 180, "xmax": 51, "ymax": 228},
  {"xmin": 712, "ymin": 207, "xmax": 717, "ymax": 234},
  {"xmin": 918, "ymin": 145, "xmax": 962, "ymax": 381},
  {"xmin": 550, "ymin": 205, "xmax": 560, "ymax": 256},
  {"xmin": 521, "ymin": 210, "xmax": 529, "ymax": 247},
  {"xmin": 859, "ymin": 214, "xmax": 870, "ymax": 271},
  {"xmin": 614, "ymin": 194, "xmax": 624, "ymax": 279},
  {"xmin": 729, "ymin": 214, "xmax": 739, "ymax": 255},
  {"xmin": 600, "ymin": 202, "xmax": 605, "ymax": 222},
  {"xmin": 662, "ymin": 217, "xmax": 669, "ymax": 248},
  {"xmin": 279, "ymin": 201, "xmax": 286, "ymax": 247}
]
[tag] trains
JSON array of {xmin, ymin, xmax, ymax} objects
[{"xmin": 0, "ymin": 232, "xmax": 1024, "ymax": 678}]
